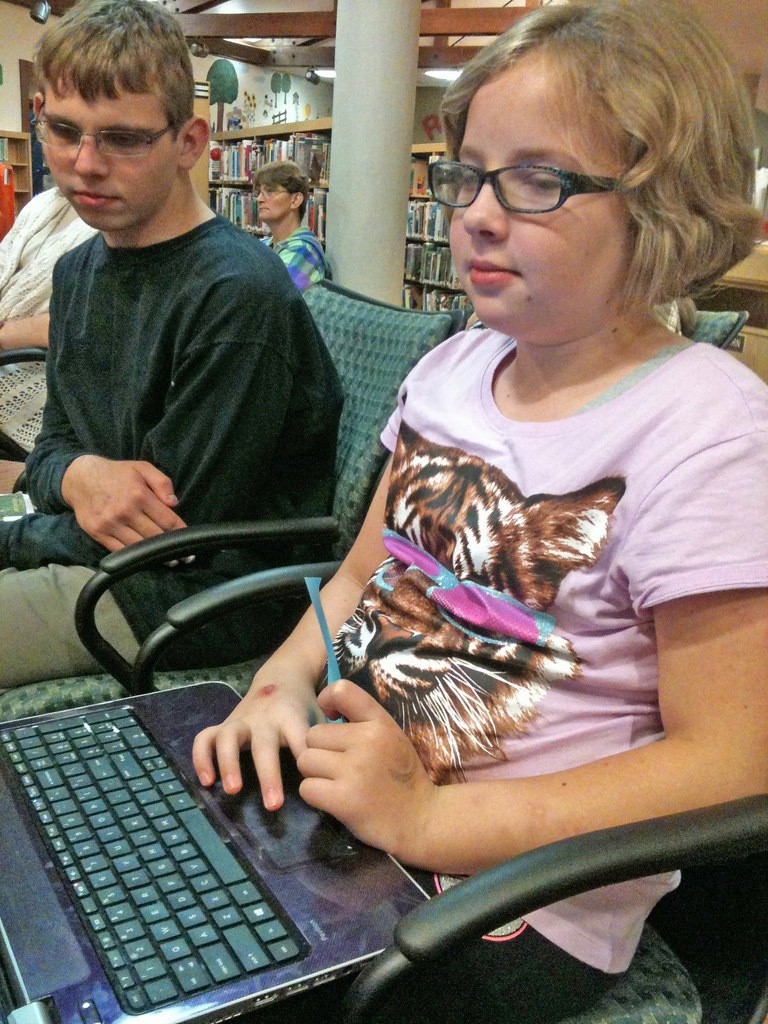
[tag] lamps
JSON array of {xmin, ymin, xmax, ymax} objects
[
  {"xmin": 304, "ymin": 71, "xmax": 320, "ymax": 85},
  {"xmin": 190, "ymin": 42, "xmax": 209, "ymax": 58},
  {"xmin": 28, "ymin": 3, "xmax": 51, "ymax": 24}
]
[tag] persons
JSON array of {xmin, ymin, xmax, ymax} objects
[
  {"xmin": 1, "ymin": 0, "xmax": 346, "ymax": 693},
  {"xmin": 194, "ymin": 0, "xmax": 768, "ymax": 1023}
]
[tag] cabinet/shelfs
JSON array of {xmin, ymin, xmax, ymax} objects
[{"xmin": 0, "ymin": 115, "xmax": 768, "ymax": 378}]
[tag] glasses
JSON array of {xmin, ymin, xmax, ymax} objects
[
  {"xmin": 252, "ymin": 186, "xmax": 288, "ymax": 198},
  {"xmin": 35, "ymin": 101, "xmax": 170, "ymax": 156},
  {"xmin": 427, "ymin": 159, "xmax": 621, "ymax": 213}
]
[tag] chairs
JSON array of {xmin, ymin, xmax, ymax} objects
[{"xmin": 0, "ymin": 279, "xmax": 768, "ymax": 1024}]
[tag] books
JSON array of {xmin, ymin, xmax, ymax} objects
[{"xmin": 209, "ymin": 133, "xmax": 471, "ymax": 321}]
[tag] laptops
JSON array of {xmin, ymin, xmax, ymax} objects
[{"xmin": 0, "ymin": 681, "xmax": 432, "ymax": 1024}]
